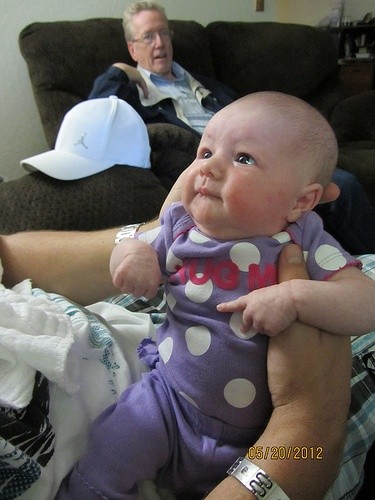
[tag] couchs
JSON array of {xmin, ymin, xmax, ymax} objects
[
  {"xmin": 18, "ymin": 10, "xmax": 375, "ymax": 269},
  {"xmin": 1, "ymin": 119, "xmax": 200, "ymax": 235}
]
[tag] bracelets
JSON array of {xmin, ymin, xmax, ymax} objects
[
  {"xmin": 226, "ymin": 457, "xmax": 292, "ymax": 500},
  {"xmin": 114, "ymin": 222, "xmax": 143, "ymax": 243}
]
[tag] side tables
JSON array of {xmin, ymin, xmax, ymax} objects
[{"xmin": 339, "ymin": 56, "xmax": 375, "ymax": 90}]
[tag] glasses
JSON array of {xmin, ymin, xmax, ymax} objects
[{"xmin": 133, "ymin": 30, "xmax": 174, "ymax": 45}]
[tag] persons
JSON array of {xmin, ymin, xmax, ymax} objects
[
  {"xmin": 59, "ymin": 93, "xmax": 375, "ymax": 500},
  {"xmin": 85, "ymin": 1, "xmax": 374, "ymax": 254},
  {"xmin": 0, "ymin": 213, "xmax": 374, "ymax": 500}
]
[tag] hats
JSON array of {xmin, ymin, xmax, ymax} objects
[{"xmin": 20, "ymin": 94, "xmax": 152, "ymax": 180}]
